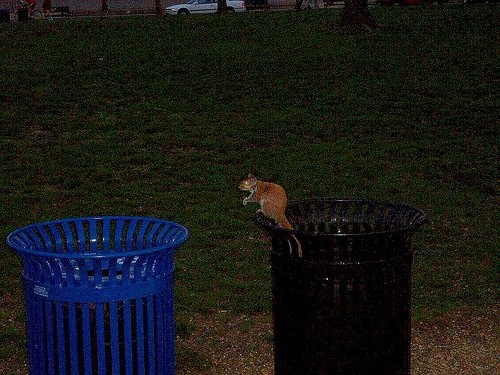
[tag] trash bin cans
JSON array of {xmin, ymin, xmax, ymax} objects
[
  {"xmin": 259, "ymin": 200, "xmax": 428, "ymax": 375},
  {"xmin": 5, "ymin": 215, "xmax": 189, "ymax": 375}
]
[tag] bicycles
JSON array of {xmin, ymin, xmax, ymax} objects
[{"xmin": 9, "ymin": 0, "xmax": 43, "ymax": 22}]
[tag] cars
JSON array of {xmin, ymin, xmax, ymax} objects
[{"xmin": 166, "ymin": 0, "xmax": 247, "ymax": 15}]
